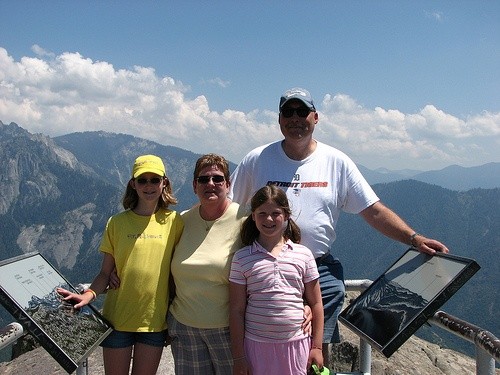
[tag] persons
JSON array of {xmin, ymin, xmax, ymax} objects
[
  {"xmin": 227, "ymin": 185, "xmax": 325, "ymax": 375},
  {"xmin": 226, "ymin": 88, "xmax": 448, "ymax": 341},
  {"xmin": 110, "ymin": 154, "xmax": 314, "ymax": 375},
  {"xmin": 57, "ymin": 155, "xmax": 184, "ymax": 375}
]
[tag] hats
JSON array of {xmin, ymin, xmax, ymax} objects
[
  {"xmin": 279, "ymin": 88, "xmax": 316, "ymax": 112},
  {"xmin": 132, "ymin": 155, "xmax": 165, "ymax": 178}
]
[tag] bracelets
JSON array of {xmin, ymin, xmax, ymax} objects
[
  {"xmin": 235, "ymin": 355, "xmax": 246, "ymax": 361},
  {"xmin": 84, "ymin": 289, "xmax": 97, "ymax": 303},
  {"xmin": 410, "ymin": 233, "xmax": 425, "ymax": 247}
]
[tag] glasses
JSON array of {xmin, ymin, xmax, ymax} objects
[
  {"xmin": 280, "ymin": 107, "xmax": 315, "ymax": 118},
  {"xmin": 133, "ymin": 176, "xmax": 165, "ymax": 185},
  {"xmin": 195, "ymin": 175, "xmax": 226, "ymax": 185}
]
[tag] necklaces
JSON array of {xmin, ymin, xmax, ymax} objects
[{"xmin": 200, "ymin": 202, "xmax": 227, "ymax": 232}]
